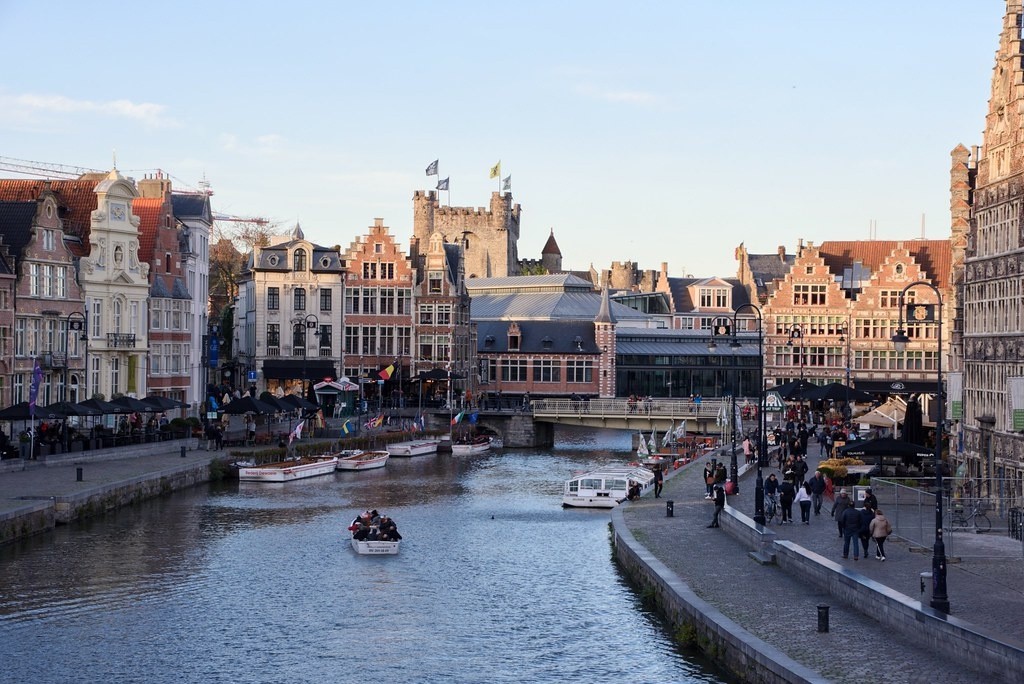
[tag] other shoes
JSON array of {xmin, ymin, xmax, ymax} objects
[
  {"xmin": 783, "ymin": 521, "xmax": 787, "ymax": 523},
  {"xmin": 715, "ymin": 525, "xmax": 719, "ymax": 527},
  {"xmin": 876, "ymin": 555, "xmax": 880, "ymax": 559},
  {"xmin": 768, "ymin": 514, "xmax": 772, "ymax": 519},
  {"xmin": 788, "ymin": 518, "xmax": 794, "ymax": 522},
  {"xmin": 802, "ymin": 520, "xmax": 809, "ymax": 525},
  {"xmin": 842, "ymin": 554, "xmax": 847, "ymax": 558},
  {"xmin": 854, "ymin": 556, "xmax": 858, "ymax": 560},
  {"xmin": 864, "ymin": 552, "xmax": 868, "ymax": 558},
  {"xmin": 707, "ymin": 525, "xmax": 714, "ymax": 528},
  {"xmin": 881, "ymin": 556, "xmax": 885, "ymax": 561}
]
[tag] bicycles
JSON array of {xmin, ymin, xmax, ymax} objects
[{"xmin": 942, "ymin": 499, "xmax": 992, "ymax": 533}]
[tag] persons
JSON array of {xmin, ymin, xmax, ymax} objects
[
  {"xmin": 689, "ymin": 393, "xmax": 702, "ymax": 413},
  {"xmin": 742, "ymin": 417, "xmax": 892, "ymax": 561},
  {"xmin": 652, "ymin": 463, "xmax": 664, "ymax": 498},
  {"xmin": 521, "ymin": 390, "xmax": 532, "ymax": 412},
  {"xmin": 0, "ymin": 411, "xmax": 256, "ymax": 461},
  {"xmin": 349, "ymin": 509, "xmax": 402, "ymax": 542},
  {"xmin": 627, "ymin": 394, "xmax": 653, "ymax": 415},
  {"xmin": 703, "ymin": 459, "xmax": 727, "ymax": 529},
  {"xmin": 571, "ymin": 392, "xmax": 590, "ymax": 413},
  {"xmin": 460, "ymin": 388, "xmax": 503, "ymax": 412},
  {"xmin": 615, "ymin": 480, "xmax": 640, "ymax": 505}
]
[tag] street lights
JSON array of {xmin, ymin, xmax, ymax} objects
[
  {"xmin": 888, "ymin": 280, "xmax": 951, "ymax": 615},
  {"xmin": 63, "ymin": 311, "xmax": 89, "ymax": 402},
  {"xmin": 302, "ymin": 313, "xmax": 321, "ymax": 400},
  {"xmin": 706, "ymin": 304, "xmax": 766, "ymax": 527},
  {"xmin": 838, "ymin": 320, "xmax": 850, "ymax": 409},
  {"xmin": 786, "ymin": 323, "xmax": 804, "ymax": 420}
]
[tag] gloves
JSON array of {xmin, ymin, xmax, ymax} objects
[{"xmin": 832, "ymin": 511, "xmax": 834, "ymax": 517}]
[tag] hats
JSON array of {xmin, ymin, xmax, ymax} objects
[
  {"xmin": 866, "ymin": 489, "xmax": 872, "ymax": 495},
  {"xmin": 840, "ymin": 489, "xmax": 846, "ymax": 493}
]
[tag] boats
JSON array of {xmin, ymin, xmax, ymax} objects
[
  {"xmin": 349, "ymin": 515, "xmax": 401, "ymax": 555},
  {"xmin": 238, "ymin": 455, "xmax": 338, "ymax": 483},
  {"xmin": 562, "ymin": 463, "xmax": 657, "ymax": 508},
  {"xmin": 437, "ymin": 433, "xmax": 454, "ymax": 452},
  {"xmin": 452, "ymin": 435, "xmax": 494, "ymax": 455},
  {"xmin": 385, "ymin": 438, "xmax": 442, "ymax": 457},
  {"xmin": 330, "ymin": 449, "xmax": 365, "ymax": 460},
  {"xmin": 626, "ymin": 434, "xmax": 721, "ymax": 476},
  {"xmin": 336, "ymin": 450, "xmax": 391, "ymax": 471}
]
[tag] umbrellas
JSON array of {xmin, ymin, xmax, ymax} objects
[
  {"xmin": 768, "ymin": 381, "xmax": 937, "ymax": 477},
  {"xmin": 0, "ymin": 396, "xmax": 191, "ymax": 460},
  {"xmin": 410, "ymin": 367, "xmax": 466, "ymax": 395},
  {"xmin": 212, "ymin": 394, "xmax": 318, "ymax": 445}
]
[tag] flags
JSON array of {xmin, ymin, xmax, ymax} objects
[
  {"xmin": 378, "ymin": 361, "xmax": 398, "ymax": 382},
  {"xmin": 451, "ymin": 411, "xmax": 479, "ymax": 425},
  {"xmin": 436, "ymin": 177, "xmax": 448, "ymax": 190},
  {"xmin": 490, "ymin": 162, "xmax": 499, "ymax": 178},
  {"xmin": 717, "ymin": 399, "xmax": 757, "ymax": 435},
  {"xmin": 502, "ymin": 176, "xmax": 511, "ymax": 190},
  {"xmin": 426, "ymin": 160, "xmax": 438, "ymax": 176},
  {"xmin": 287, "ymin": 409, "xmax": 425, "ymax": 445},
  {"xmin": 637, "ymin": 423, "xmax": 685, "ymax": 458}
]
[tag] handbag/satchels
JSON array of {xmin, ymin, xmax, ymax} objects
[
  {"xmin": 707, "ymin": 477, "xmax": 714, "ymax": 484},
  {"xmin": 749, "ymin": 440, "xmax": 753, "ymax": 451},
  {"xmin": 778, "ymin": 454, "xmax": 782, "ymax": 461},
  {"xmin": 658, "ymin": 480, "xmax": 663, "ymax": 484}
]
